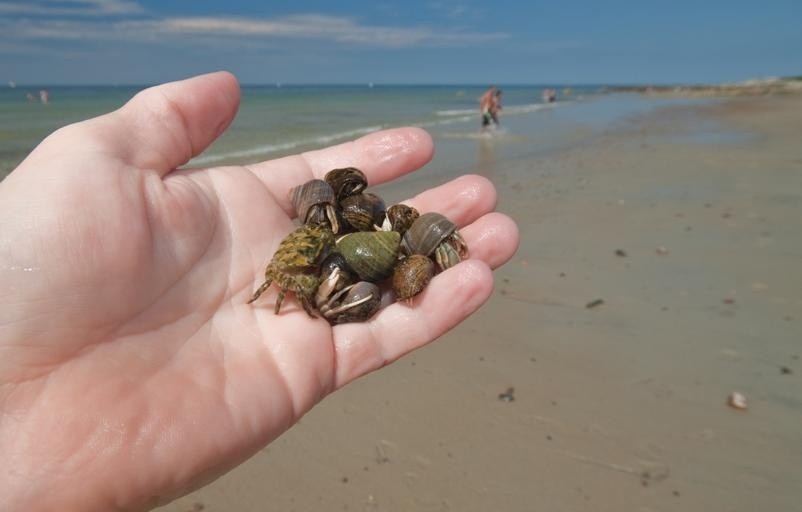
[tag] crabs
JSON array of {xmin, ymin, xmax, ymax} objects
[{"xmin": 244, "ymin": 219, "xmax": 337, "ymax": 321}]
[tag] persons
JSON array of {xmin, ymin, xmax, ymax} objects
[
  {"xmin": 493, "ymin": 92, "xmax": 503, "ymax": 115},
  {"xmin": 480, "ymin": 85, "xmax": 498, "ymax": 133},
  {"xmin": 1, "ymin": 69, "xmax": 523, "ymax": 512}
]
